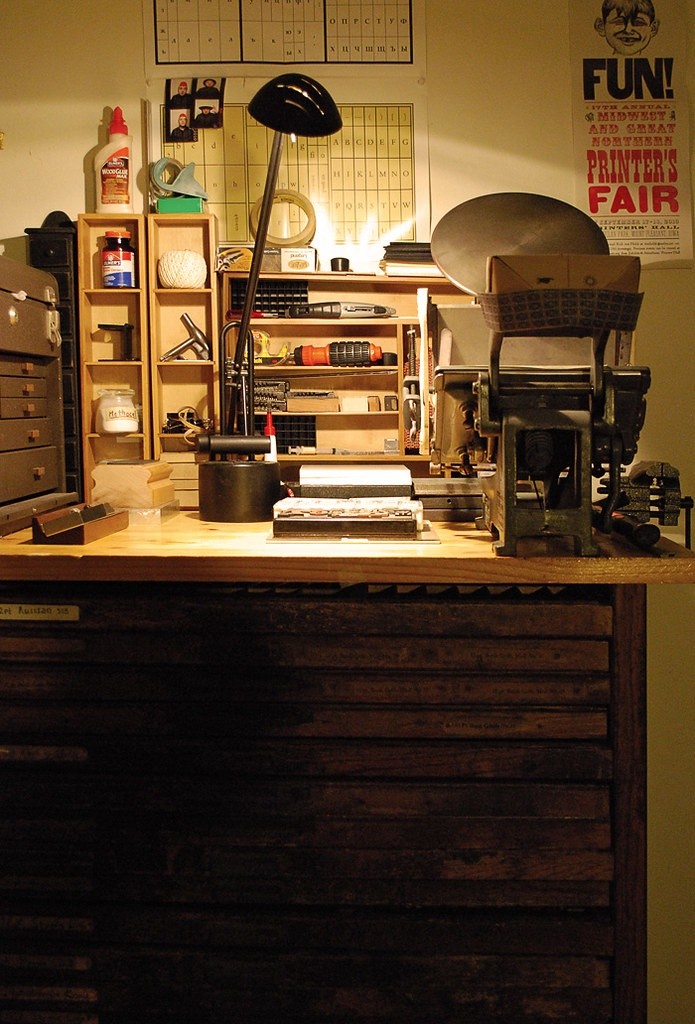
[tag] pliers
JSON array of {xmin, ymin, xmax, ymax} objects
[{"xmin": 289, "ymin": 302, "xmax": 397, "ymax": 319}]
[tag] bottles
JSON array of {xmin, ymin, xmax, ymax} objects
[
  {"xmin": 92, "ymin": 105, "xmax": 135, "ymax": 214},
  {"xmin": 92, "ymin": 384, "xmax": 139, "ymax": 434},
  {"xmin": 101, "ymin": 228, "xmax": 136, "ymax": 289}
]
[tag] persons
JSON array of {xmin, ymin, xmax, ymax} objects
[{"xmin": 170, "ymin": 78, "xmax": 220, "ymax": 142}]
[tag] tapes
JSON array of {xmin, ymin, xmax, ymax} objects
[{"xmin": 249, "ymin": 189, "xmax": 316, "ymax": 250}]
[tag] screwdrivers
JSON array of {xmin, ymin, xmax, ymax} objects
[{"xmin": 255, "ymin": 341, "xmax": 383, "ymax": 368}]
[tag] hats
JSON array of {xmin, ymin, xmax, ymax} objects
[
  {"xmin": 179, "ymin": 113, "xmax": 186, "ymax": 118},
  {"xmin": 203, "ymin": 78, "xmax": 216, "ymax": 86},
  {"xmin": 199, "ymin": 104, "xmax": 214, "ymax": 110},
  {"xmin": 180, "ymin": 81, "xmax": 187, "ymax": 86}
]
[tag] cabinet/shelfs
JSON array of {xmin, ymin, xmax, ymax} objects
[
  {"xmin": 78, "ymin": 214, "xmax": 151, "ymax": 511},
  {"xmin": 148, "ymin": 209, "xmax": 221, "ymax": 508},
  {"xmin": 220, "ymin": 265, "xmax": 475, "ymax": 488}
]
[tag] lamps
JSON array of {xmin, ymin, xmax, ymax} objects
[{"xmin": 196, "ymin": 73, "xmax": 344, "ymax": 525}]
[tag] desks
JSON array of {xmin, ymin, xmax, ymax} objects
[{"xmin": 1, "ymin": 505, "xmax": 695, "ymax": 1023}]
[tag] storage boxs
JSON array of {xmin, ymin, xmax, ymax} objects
[
  {"xmin": 0, "ymin": 252, "xmax": 78, "ymax": 537},
  {"xmin": 299, "ymin": 464, "xmax": 413, "ymax": 500}
]
